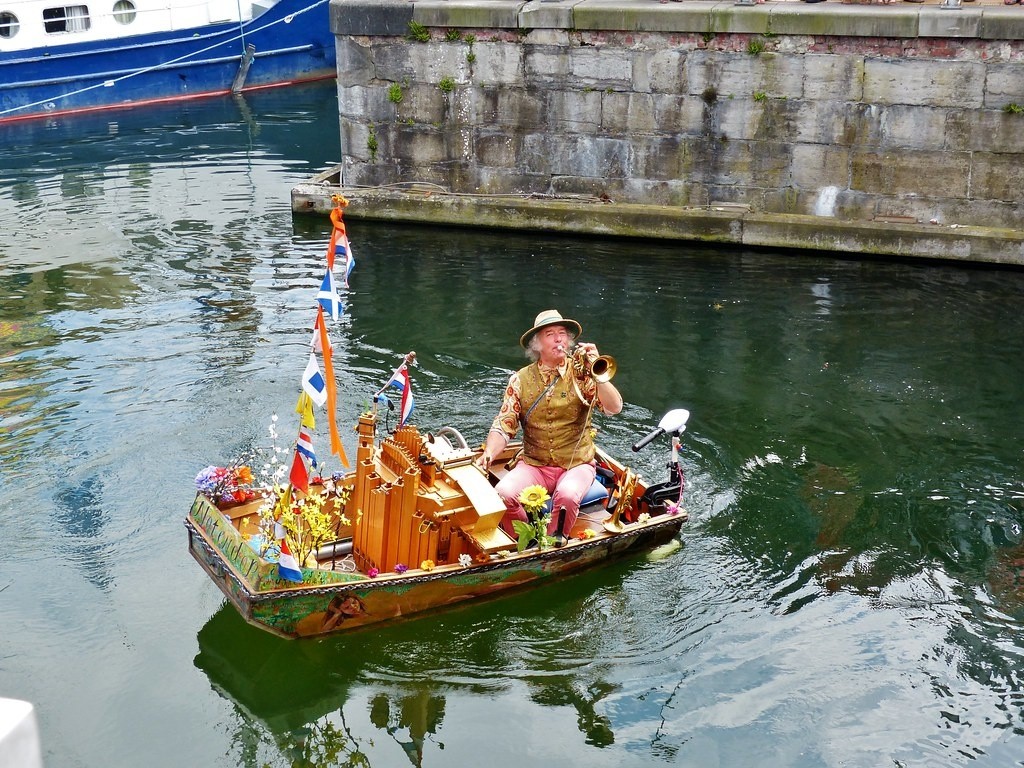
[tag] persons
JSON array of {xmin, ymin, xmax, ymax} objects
[{"xmin": 475, "ymin": 310, "xmax": 622, "ymax": 552}]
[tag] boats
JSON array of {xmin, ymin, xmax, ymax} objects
[
  {"xmin": 1, "ymin": 0, "xmax": 334, "ymax": 135},
  {"xmin": 183, "ymin": 190, "xmax": 694, "ymax": 642}
]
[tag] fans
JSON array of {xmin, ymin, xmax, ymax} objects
[{"xmin": 667, "ymin": 504, "xmax": 681, "ymax": 517}]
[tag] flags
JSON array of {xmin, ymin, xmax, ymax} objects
[{"xmin": 274, "ymin": 207, "xmax": 414, "ymax": 583}]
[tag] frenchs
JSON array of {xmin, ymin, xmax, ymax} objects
[{"xmin": 601, "ymin": 467, "xmax": 643, "ymax": 533}]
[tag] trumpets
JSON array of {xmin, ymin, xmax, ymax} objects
[{"xmin": 557, "ymin": 344, "xmax": 618, "ymax": 384}]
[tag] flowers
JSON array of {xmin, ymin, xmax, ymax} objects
[
  {"xmin": 243, "ymin": 484, "xmax": 370, "ymax": 572},
  {"xmin": 457, "ymin": 553, "xmax": 473, "ymax": 567},
  {"xmin": 638, "ymin": 513, "xmax": 651, "ymax": 525},
  {"xmin": 421, "ymin": 560, "xmax": 435, "ymax": 572},
  {"xmin": 495, "ymin": 549, "xmax": 510, "ymax": 559},
  {"xmin": 511, "ymin": 485, "xmax": 558, "ymax": 554},
  {"xmin": 252, "ymin": 414, "xmax": 292, "ymax": 545},
  {"xmin": 475, "ymin": 552, "xmax": 492, "ymax": 563},
  {"xmin": 577, "ymin": 530, "xmax": 599, "ymax": 540},
  {"xmin": 394, "ymin": 563, "xmax": 408, "ymax": 574},
  {"xmin": 368, "ymin": 567, "xmax": 379, "ymax": 579},
  {"xmin": 197, "ymin": 436, "xmax": 258, "ymax": 508}
]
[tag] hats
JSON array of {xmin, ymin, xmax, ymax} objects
[{"xmin": 520, "ymin": 309, "xmax": 582, "ymax": 347}]
